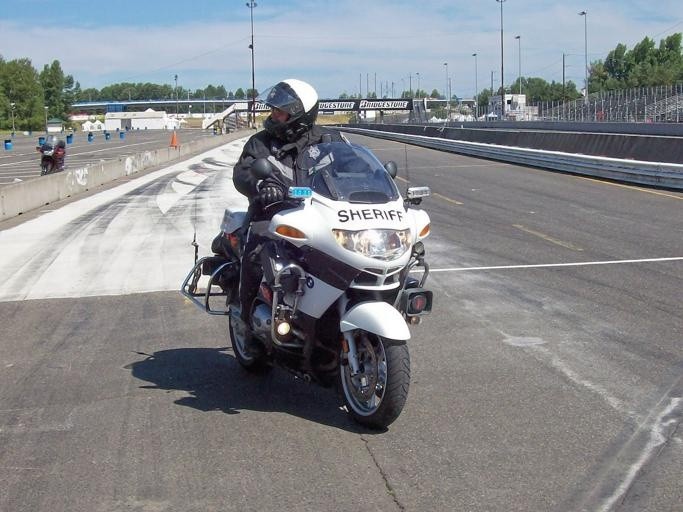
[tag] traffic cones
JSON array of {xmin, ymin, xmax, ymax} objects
[{"xmin": 169, "ymin": 130, "xmax": 179, "ymax": 147}]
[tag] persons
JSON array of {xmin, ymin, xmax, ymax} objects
[{"xmin": 232, "ymin": 79, "xmax": 350, "ymax": 356}]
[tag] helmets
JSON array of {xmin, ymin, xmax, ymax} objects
[{"xmin": 256, "ymin": 79, "xmax": 320, "ymax": 139}]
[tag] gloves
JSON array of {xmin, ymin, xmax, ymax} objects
[{"xmin": 258, "ymin": 180, "xmax": 284, "ymax": 206}]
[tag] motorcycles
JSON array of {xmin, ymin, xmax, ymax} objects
[
  {"xmin": 35, "ymin": 134, "xmax": 67, "ymax": 178},
  {"xmin": 176, "ymin": 141, "xmax": 436, "ymax": 430}
]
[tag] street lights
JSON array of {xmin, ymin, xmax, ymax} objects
[
  {"xmin": 443, "ymin": 62, "xmax": 450, "ymax": 121},
  {"xmin": 10, "ymin": 102, "xmax": 17, "ymax": 135},
  {"xmin": 243, "ymin": 0, "xmax": 261, "ymax": 127},
  {"xmin": 514, "ymin": 35, "xmax": 522, "ymax": 95},
  {"xmin": 415, "ymin": 72, "xmax": 421, "ymax": 100},
  {"xmin": 172, "ymin": 75, "xmax": 179, "ymax": 119},
  {"xmin": 578, "ymin": 9, "xmax": 590, "ymax": 105},
  {"xmin": 496, "ymin": 0, "xmax": 510, "ymax": 120},
  {"xmin": 471, "ymin": 53, "xmax": 480, "ymax": 118},
  {"xmin": 43, "ymin": 105, "xmax": 51, "ymax": 135}
]
